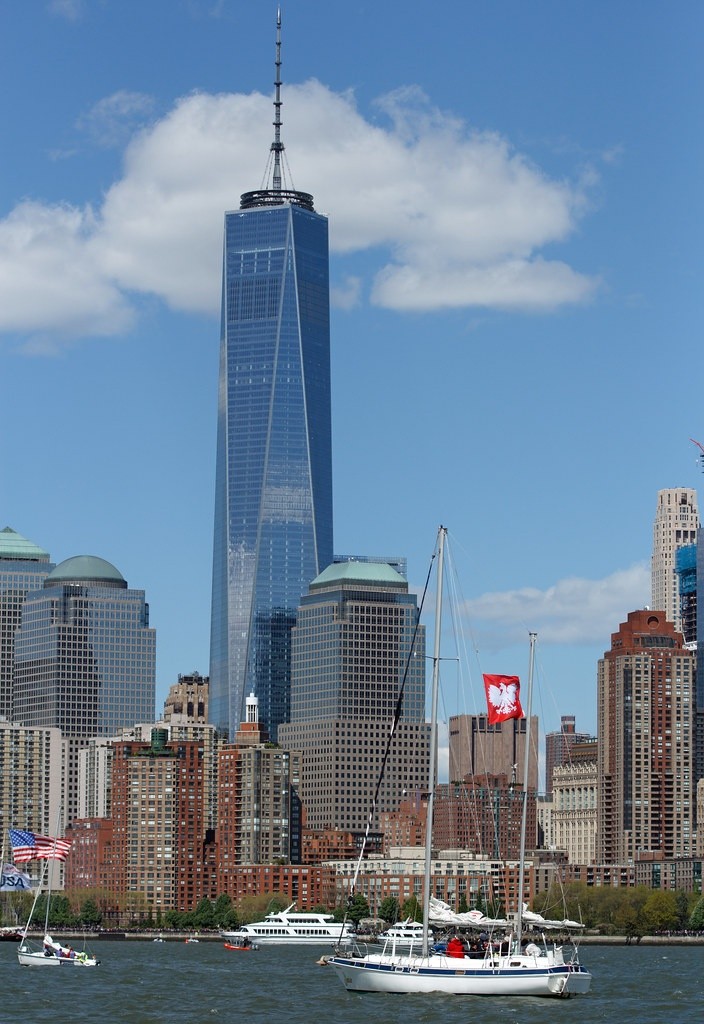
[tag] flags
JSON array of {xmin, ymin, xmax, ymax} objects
[
  {"xmin": 483, "ymin": 673, "xmax": 526, "ymax": 725},
  {"xmin": 9, "ymin": 829, "xmax": 71, "ymax": 862},
  {"xmin": 0, "ymin": 863, "xmax": 31, "ymax": 891}
]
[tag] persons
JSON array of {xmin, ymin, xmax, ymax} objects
[
  {"xmin": 67, "ymin": 949, "xmax": 75, "ymax": 958},
  {"xmin": 57, "ymin": 948, "xmax": 63, "ymax": 957},
  {"xmin": 79, "ymin": 951, "xmax": 87, "ymax": 960},
  {"xmin": 44, "ymin": 946, "xmax": 54, "ymax": 956},
  {"xmin": 447, "ymin": 938, "xmax": 464, "ymax": 958},
  {"xmin": 243, "ymin": 936, "xmax": 247, "ymax": 948},
  {"xmin": 469, "ymin": 943, "xmax": 477, "ymax": 959}
]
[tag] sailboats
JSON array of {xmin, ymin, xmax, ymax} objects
[
  {"xmin": 314, "ymin": 520, "xmax": 596, "ymax": 999},
  {"xmin": 15, "ymin": 803, "xmax": 102, "ymax": 967}
]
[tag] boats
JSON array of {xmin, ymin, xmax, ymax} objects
[
  {"xmin": 223, "ymin": 941, "xmax": 259, "ymax": 951},
  {"xmin": 239, "ymin": 899, "xmax": 359, "ymax": 947},
  {"xmin": 376, "ymin": 916, "xmax": 436, "ymax": 947}
]
